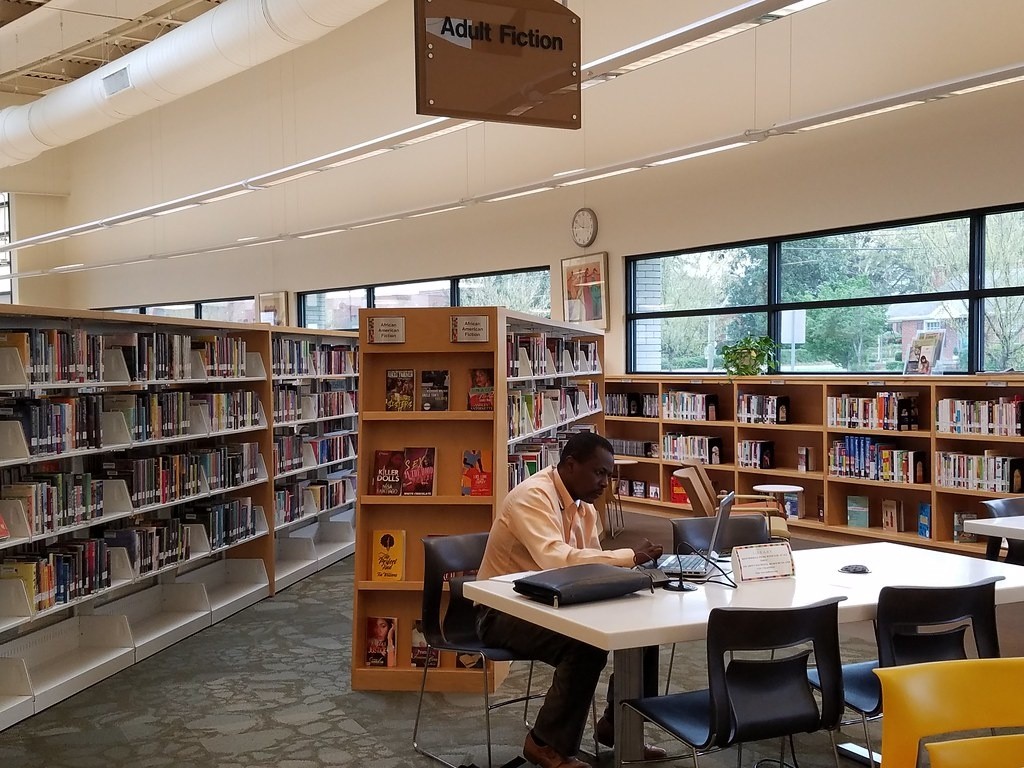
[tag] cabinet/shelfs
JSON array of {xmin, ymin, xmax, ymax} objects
[
  {"xmin": 349, "ymin": 306, "xmax": 613, "ymax": 702},
  {"xmin": 606, "ymin": 370, "xmax": 1024, "ymax": 545},
  {"xmin": 270, "ymin": 325, "xmax": 360, "ymax": 596},
  {"xmin": 0, "ymin": 301, "xmax": 275, "ymax": 732}
]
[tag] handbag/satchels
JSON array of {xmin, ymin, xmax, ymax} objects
[{"xmin": 512, "ymin": 563, "xmax": 654, "ymax": 609}]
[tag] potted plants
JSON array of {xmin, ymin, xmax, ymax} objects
[{"xmin": 717, "ymin": 332, "xmax": 782, "ymax": 383}]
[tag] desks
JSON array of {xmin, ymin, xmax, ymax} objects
[
  {"xmin": 751, "ymin": 485, "xmax": 803, "ymax": 520},
  {"xmin": 964, "ymin": 514, "xmax": 1024, "ymax": 560},
  {"xmin": 462, "ymin": 542, "xmax": 1024, "ymax": 768}
]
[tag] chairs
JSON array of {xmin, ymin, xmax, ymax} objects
[
  {"xmin": 663, "ymin": 514, "xmax": 776, "ymax": 696},
  {"xmin": 978, "ymin": 496, "xmax": 1024, "ymax": 567},
  {"xmin": 672, "ymin": 458, "xmax": 793, "ymax": 552},
  {"xmin": 617, "ymin": 574, "xmax": 1024, "ymax": 768},
  {"xmin": 410, "ymin": 530, "xmax": 601, "ymax": 768}
]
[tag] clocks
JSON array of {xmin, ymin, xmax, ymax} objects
[{"xmin": 570, "ymin": 206, "xmax": 598, "ymax": 248}]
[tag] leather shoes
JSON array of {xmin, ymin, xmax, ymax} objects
[
  {"xmin": 523, "ymin": 729, "xmax": 593, "ymax": 768},
  {"xmin": 594, "ymin": 716, "xmax": 667, "ymax": 760}
]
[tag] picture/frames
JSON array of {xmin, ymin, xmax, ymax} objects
[{"xmin": 559, "ymin": 249, "xmax": 610, "ymax": 334}]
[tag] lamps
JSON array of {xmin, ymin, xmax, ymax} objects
[{"xmin": 0, "ymin": 0, "xmax": 1024, "ymax": 280}]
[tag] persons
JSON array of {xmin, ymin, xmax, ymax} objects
[
  {"xmin": 411, "ymin": 620, "xmax": 428, "ymax": 647},
  {"xmin": 472, "ymin": 433, "xmax": 668, "ymax": 768},
  {"xmin": 368, "ymin": 618, "xmax": 396, "ymax": 667},
  {"xmin": 470, "ymin": 369, "xmax": 494, "ymax": 388}
]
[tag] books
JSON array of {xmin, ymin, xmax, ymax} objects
[
  {"xmin": 374, "ymin": 449, "xmax": 403, "ymax": 495},
  {"xmin": 466, "ymin": 368, "xmax": 494, "ymax": 412},
  {"xmin": 404, "ymin": 446, "xmax": 438, "ymax": 497},
  {"xmin": 0, "ymin": 328, "xmax": 360, "ymax": 619},
  {"xmin": 421, "ymin": 370, "xmax": 451, "ymax": 411},
  {"xmin": 456, "ymin": 652, "xmax": 486, "ymax": 669},
  {"xmin": 366, "ymin": 616, "xmax": 398, "ymax": 667},
  {"xmin": 386, "ymin": 369, "xmax": 416, "ymax": 411},
  {"xmin": 461, "ymin": 449, "xmax": 492, "ymax": 496},
  {"xmin": 411, "ymin": 619, "xmax": 441, "ymax": 668},
  {"xmin": 372, "ymin": 529, "xmax": 407, "ymax": 581},
  {"xmin": 507, "ymin": 328, "xmax": 1024, "ymax": 548}
]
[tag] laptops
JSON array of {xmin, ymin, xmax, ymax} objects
[{"xmin": 637, "ymin": 490, "xmax": 735, "ymax": 577}]
